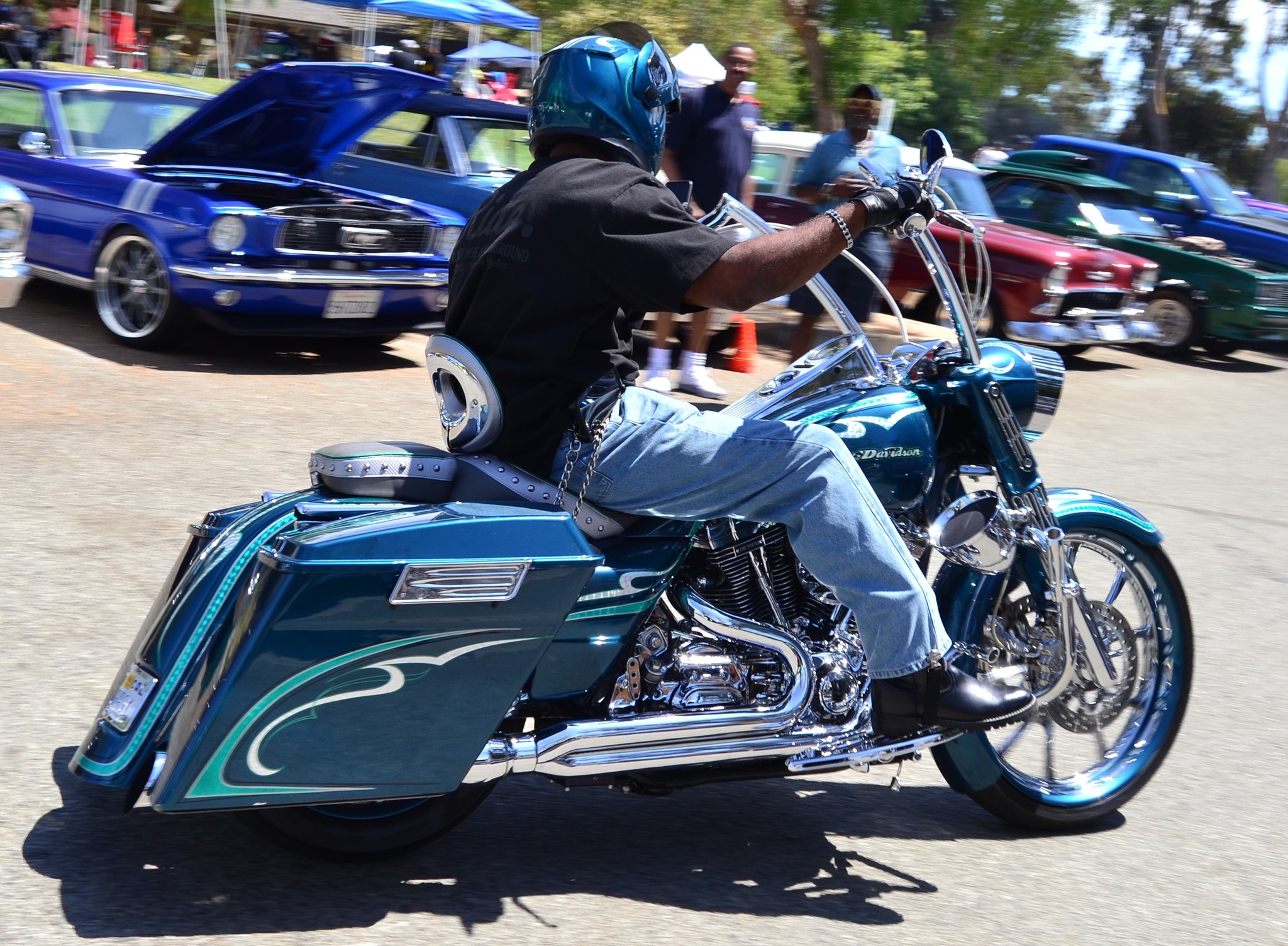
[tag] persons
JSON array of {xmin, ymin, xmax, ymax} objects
[
  {"xmin": 383, "ymin": 38, "xmax": 516, "ymax": 102},
  {"xmin": 446, "ymin": 21, "xmax": 1040, "ymax": 741},
  {"xmin": 2, "ymin": 0, "xmax": 122, "ymax": 71},
  {"xmin": 643, "ymin": 40, "xmax": 762, "ymax": 402},
  {"xmin": 786, "ymin": 82, "xmax": 910, "ymax": 377}
]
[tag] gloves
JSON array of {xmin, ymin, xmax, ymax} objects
[{"xmin": 849, "ymin": 180, "xmax": 937, "ymax": 233}]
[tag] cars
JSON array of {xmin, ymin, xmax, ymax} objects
[
  {"xmin": 748, "ymin": 127, "xmax": 1288, "ymax": 361},
  {"xmin": 0, "ymin": 0, "xmax": 542, "ymax": 349}
]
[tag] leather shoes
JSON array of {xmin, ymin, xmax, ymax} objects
[{"xmin": 871, "ymin": 667, "xmax": 1035, "ymax": 744}]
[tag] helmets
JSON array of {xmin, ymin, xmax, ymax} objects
[{"xmin": 523, "ymin": 20, "xmax": 682, "ymax": 173}]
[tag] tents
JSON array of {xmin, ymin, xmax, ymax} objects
[
  {"xmin": 299, "ymin": 0, "xmax": 542, "ymax": 102},
  {"xmin": 663, "ymin": 41, "xmax": 759, "ymax": 120}
]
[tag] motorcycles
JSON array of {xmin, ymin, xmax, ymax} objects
[{"xmin": 66, "ymin": 130, "xmax": 1195, "ymax": 886}]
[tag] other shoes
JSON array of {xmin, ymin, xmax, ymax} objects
[
  {"xmin": 678, "ymin": 370, "xmax": 726, "ymax": 399},
  {"xmin": 643, "ymin": 376, "xmax": 671, "ymax": 394}
]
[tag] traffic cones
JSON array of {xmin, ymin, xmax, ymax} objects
[{"xmin": 732, "ymin": 320, "xmax": 759, "ymax": 353}]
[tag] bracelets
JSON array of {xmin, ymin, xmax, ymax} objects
[{"xmin": 824, "ymin": 207, "xmax": 854, "ymax": 251}]
